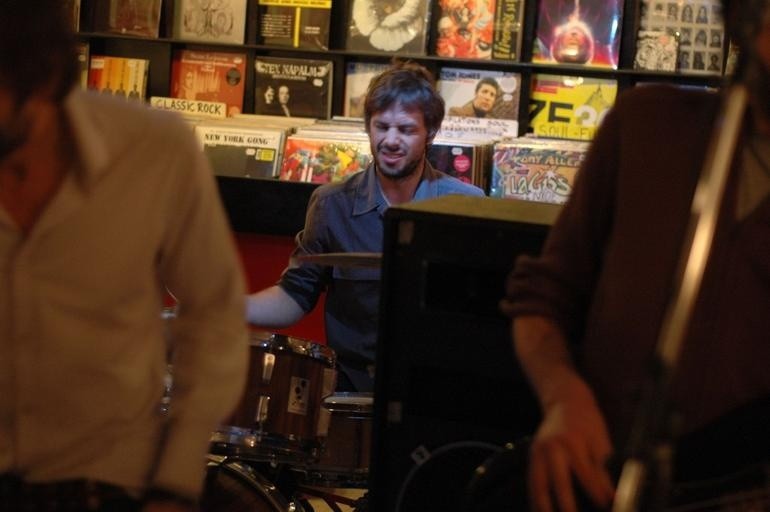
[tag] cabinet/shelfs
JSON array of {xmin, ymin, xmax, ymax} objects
[{"xmin": 62, "ymin": 0, "xmax": 741, "ymax": 234}]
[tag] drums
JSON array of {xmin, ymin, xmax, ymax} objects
[
  {"xmin": 319, "ymin": 391, "xmax": 375, "ymax": 487},
  {"xmin": 209, "ymin": 425, "xmax": 271, "ymax": 465},
  {"xmin": 212, "ymin": 333, "xmax": 334, "ymax": 473},
  {"xmin": 203, "ymin": 456, "xmax": 313, "ymax": 512}
]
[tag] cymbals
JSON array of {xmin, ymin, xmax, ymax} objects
[{"xmin": 295, "ymin": 251, "xmax": 383, "ymax": 268}]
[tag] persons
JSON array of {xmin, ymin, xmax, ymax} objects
[
  {"xmin": 243, "ymin": 58, "xmax": 488, "ymax": 393},
  {"xmin": 0, "ymin": 0, "xmax": 249, "ymax": 512},
  {"xmin": 501, "ymin": 1, "xmax": 769, "ymax": 510},
  {"xmin": 260, "ymin": 84, "xmax": 292, "ymax": 116},
  {"xmin": 448, "ymin": 77, "xmax": 501, "ymax": 118},
  {"xmin": 212, "ymin": 12, "xmax": 227, "ymax": 33},
  {"xmin": 631, "ymin": 2, "xmax": 743, "ymax": 82},
  {"xmin": 90, "ymin": 80, "xmax": 140, "ymax": 103}
]
